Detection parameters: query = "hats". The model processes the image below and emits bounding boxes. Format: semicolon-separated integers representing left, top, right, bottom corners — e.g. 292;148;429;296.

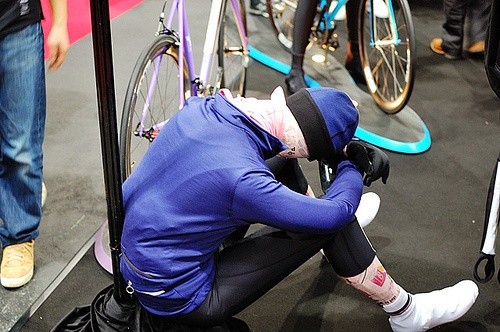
286;86;360;163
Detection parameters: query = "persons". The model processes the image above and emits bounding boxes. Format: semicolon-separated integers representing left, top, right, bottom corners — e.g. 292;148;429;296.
120;87;479;332
285;0;493;95
0;0;71;288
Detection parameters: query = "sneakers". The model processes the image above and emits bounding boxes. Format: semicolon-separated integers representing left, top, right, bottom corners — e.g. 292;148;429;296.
388;280;479;332
467;41;486;52
0;240;34;288
431;38;457;60
354;192;380;227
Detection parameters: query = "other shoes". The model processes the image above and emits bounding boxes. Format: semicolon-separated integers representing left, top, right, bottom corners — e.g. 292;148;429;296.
342;41;369;86
285;51;312;93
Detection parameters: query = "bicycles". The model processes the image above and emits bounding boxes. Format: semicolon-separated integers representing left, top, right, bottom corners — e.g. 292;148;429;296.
266;0;416;114
118;0;250;184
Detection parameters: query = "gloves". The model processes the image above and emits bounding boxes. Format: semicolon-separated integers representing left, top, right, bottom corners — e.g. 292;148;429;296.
347;139;390;186
